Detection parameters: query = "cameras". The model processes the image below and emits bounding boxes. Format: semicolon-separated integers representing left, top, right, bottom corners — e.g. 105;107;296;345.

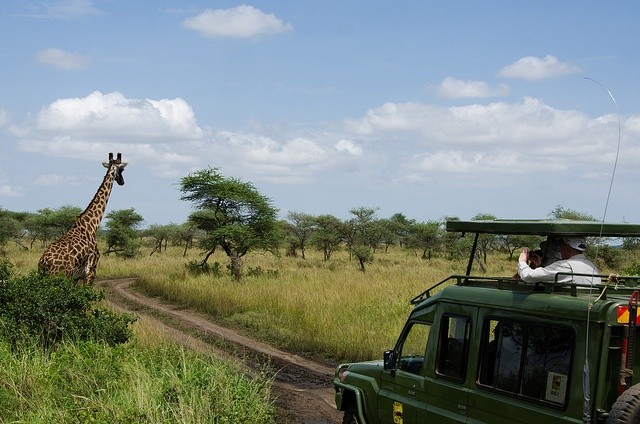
527;250;540;267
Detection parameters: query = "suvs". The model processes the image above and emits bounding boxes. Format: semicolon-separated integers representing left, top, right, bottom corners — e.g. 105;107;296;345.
332;76;640;424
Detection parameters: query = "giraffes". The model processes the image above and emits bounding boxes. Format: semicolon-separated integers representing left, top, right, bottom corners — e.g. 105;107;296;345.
38;153;129;285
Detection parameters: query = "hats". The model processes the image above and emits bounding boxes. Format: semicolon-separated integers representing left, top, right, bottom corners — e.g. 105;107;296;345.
552;235;587;252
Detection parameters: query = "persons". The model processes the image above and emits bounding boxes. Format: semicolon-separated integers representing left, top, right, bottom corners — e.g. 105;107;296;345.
516;235;601;290
